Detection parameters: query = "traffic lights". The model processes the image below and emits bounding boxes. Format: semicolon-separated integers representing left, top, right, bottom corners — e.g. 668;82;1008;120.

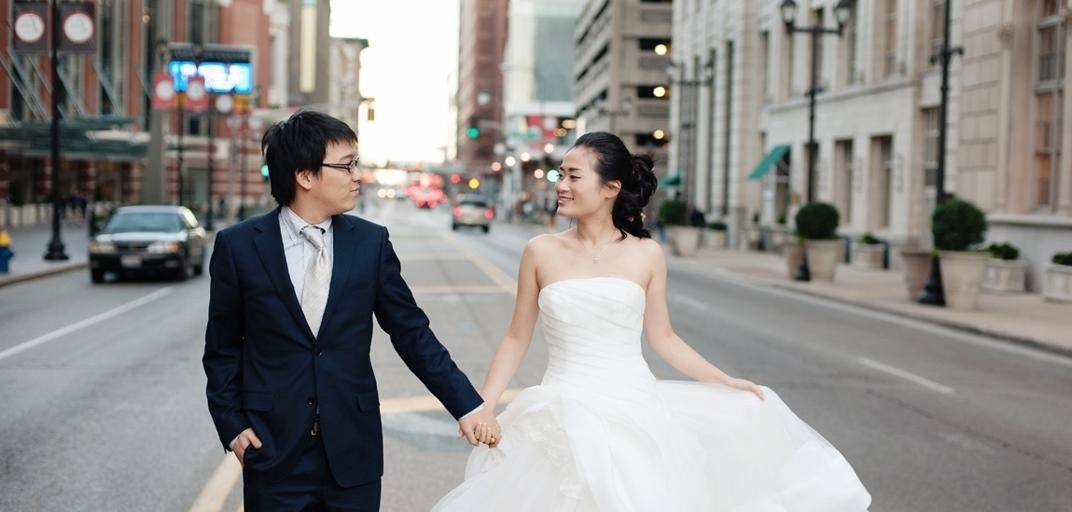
466;116;480;138
260;159;269;183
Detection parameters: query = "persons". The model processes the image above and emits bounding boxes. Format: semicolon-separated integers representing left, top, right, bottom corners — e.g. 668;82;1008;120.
203;111;495;512
431;131;870;512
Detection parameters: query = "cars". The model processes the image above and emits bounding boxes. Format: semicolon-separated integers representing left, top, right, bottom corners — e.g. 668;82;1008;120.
451;192;491;233
90;203;206;288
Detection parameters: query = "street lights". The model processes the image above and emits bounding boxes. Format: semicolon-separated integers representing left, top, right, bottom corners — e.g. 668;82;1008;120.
14;0;95;261
156;69;258;228
664;59;718;227
779;2;855;199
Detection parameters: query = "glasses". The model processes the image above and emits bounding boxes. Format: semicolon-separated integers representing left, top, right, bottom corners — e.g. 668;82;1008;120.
321;156;360;173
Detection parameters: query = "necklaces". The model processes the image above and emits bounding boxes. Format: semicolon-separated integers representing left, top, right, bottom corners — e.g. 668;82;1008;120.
573;230;623;267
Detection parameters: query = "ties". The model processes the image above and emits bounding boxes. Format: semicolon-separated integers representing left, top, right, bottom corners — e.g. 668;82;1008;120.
301;225;333;340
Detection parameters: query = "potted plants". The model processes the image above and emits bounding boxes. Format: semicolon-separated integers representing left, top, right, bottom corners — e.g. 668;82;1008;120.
972;238;1031;296
655;200;728;258
853;233;890;273
929;193;994;308
794;198;847;285
1039;251;1072;303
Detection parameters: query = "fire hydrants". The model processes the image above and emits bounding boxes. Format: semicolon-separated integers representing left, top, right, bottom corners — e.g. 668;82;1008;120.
0;230;15;276
